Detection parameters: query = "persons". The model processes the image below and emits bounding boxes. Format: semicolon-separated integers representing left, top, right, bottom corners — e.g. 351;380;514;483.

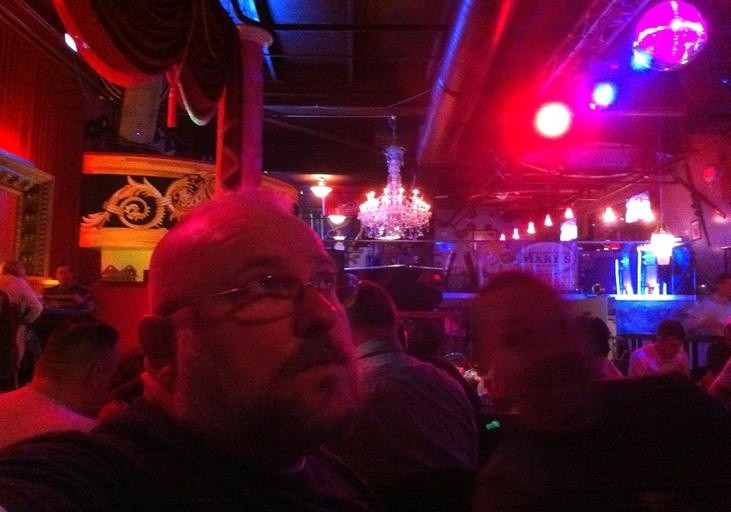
0;254;119;447
348;268;731;512
87;191;371;510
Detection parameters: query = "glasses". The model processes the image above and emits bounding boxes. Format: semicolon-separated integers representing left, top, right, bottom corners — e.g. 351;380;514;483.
152;269;363;326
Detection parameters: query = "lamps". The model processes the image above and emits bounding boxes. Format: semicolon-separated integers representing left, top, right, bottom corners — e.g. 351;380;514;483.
329;214;346;226
499;170;676;266
358;116;432;241
310;173;332;199
